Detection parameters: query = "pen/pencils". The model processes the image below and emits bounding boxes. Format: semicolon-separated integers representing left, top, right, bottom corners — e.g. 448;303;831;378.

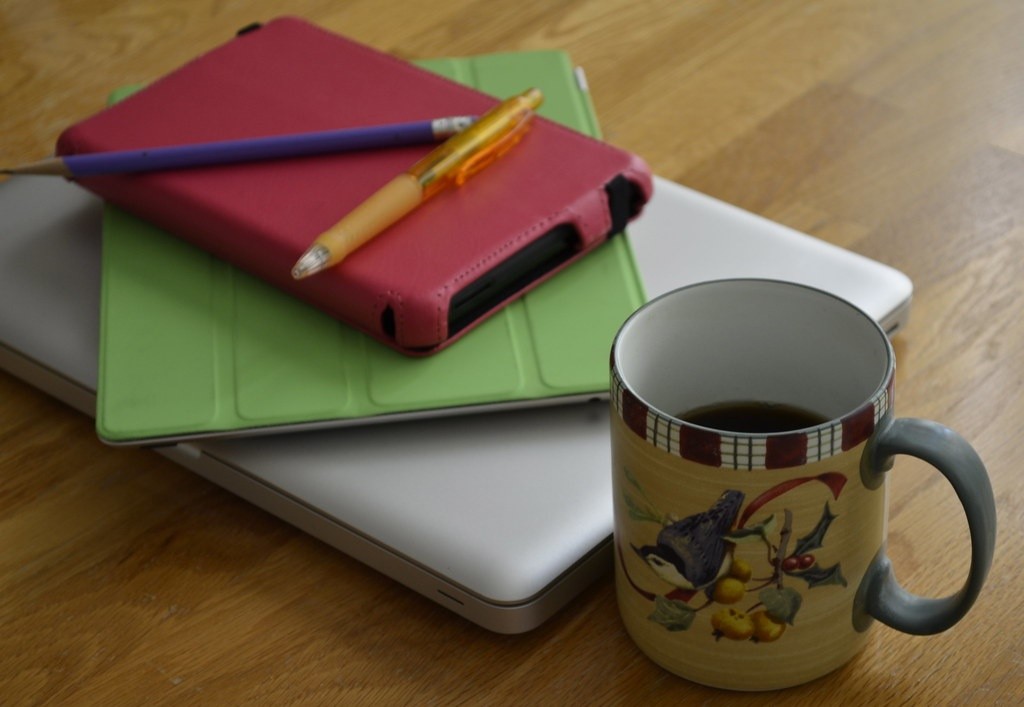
286;83;549;283
0;111;481;180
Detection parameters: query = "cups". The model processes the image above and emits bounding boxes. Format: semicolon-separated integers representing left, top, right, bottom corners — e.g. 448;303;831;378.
610;277;998;693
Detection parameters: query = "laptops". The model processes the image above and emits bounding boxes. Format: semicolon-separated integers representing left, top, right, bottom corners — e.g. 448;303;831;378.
1;172;913;634
